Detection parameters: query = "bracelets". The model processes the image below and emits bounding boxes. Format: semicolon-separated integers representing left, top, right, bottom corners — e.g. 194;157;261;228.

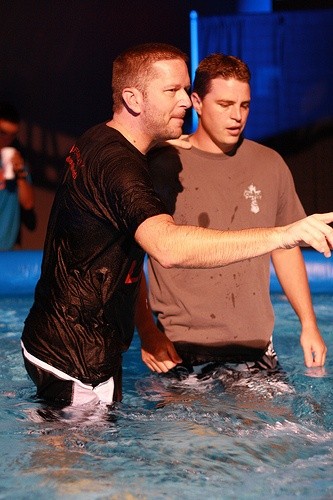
16;167;28;180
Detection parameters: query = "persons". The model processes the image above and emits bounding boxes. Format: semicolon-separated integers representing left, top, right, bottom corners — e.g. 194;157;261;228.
134;53;327;374
0;119;37;251
21;43;333;411
